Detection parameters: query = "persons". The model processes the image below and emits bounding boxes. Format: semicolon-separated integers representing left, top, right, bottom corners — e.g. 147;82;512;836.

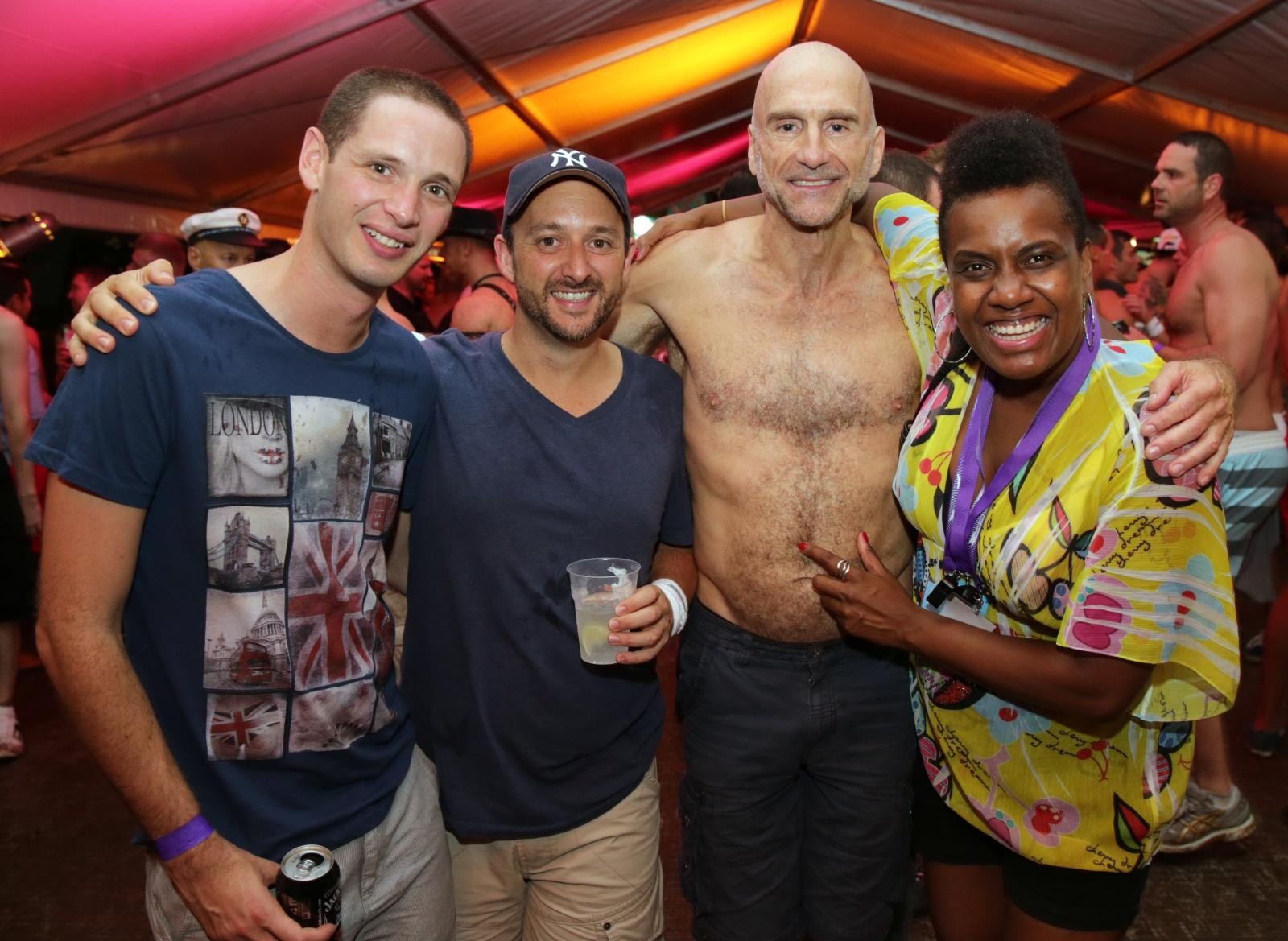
376;209;518;334
69;149;700;941
596;41;1238;941
23;67;474;941
1087;130;1288;854
634;106;1241;941
178;208;267;271
125;231;187;278
870;148;942;211
68;269;112;314
0;265;52;706
212;396;290;496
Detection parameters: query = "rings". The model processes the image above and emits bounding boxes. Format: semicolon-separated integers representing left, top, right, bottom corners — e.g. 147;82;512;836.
835;560;851;580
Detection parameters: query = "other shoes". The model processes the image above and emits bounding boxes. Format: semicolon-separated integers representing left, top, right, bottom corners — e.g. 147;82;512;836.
1241;634;1263;662
1249;725;1284;756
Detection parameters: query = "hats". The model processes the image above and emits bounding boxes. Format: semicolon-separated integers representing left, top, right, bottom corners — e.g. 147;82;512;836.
501;151;632;248
180;207;268;248
1151;228;1181;252
435;204;499;247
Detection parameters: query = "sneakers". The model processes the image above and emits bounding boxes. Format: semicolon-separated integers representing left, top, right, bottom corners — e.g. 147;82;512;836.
1157;782;1257;854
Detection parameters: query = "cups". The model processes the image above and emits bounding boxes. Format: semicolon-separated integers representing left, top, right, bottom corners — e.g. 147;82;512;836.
566;558;641;665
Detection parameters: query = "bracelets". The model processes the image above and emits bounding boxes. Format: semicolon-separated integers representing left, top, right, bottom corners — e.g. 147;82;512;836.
651;578;688;637
153;813;214;862
1154;343;1164;353
722;200;726;223
1146;317;1164;337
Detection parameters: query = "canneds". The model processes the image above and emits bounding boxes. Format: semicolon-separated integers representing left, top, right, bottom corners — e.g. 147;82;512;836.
275;843;341;941
